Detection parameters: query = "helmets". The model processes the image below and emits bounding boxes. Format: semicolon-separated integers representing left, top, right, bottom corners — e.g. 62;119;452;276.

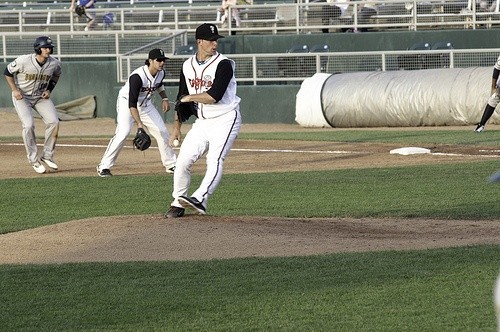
34;36;55;55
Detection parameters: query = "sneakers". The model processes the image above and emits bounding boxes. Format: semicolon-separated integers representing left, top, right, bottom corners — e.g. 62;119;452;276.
166;167;175;173
96;166;112;177
32;157;58;173
165;195;206;218
475;123;484;133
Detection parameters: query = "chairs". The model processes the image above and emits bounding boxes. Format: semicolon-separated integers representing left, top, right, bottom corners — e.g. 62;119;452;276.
169;0;457;78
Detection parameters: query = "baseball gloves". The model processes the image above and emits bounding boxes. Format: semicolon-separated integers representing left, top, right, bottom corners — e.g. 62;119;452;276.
175;94;194;123
132;128;151;151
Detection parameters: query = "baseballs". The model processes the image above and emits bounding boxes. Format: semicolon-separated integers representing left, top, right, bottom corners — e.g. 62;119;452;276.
173;140;179;147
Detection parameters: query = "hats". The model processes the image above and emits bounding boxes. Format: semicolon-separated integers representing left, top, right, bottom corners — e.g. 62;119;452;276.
195;23;225;41
149;48;169;61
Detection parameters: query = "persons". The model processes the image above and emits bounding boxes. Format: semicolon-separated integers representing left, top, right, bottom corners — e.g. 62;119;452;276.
70;0;99;38
95;49;178;179
220;0;242;30
474;54;500;132
361;0;378;25
164;23;242;218
4;36;64;175
321;0;351;33
